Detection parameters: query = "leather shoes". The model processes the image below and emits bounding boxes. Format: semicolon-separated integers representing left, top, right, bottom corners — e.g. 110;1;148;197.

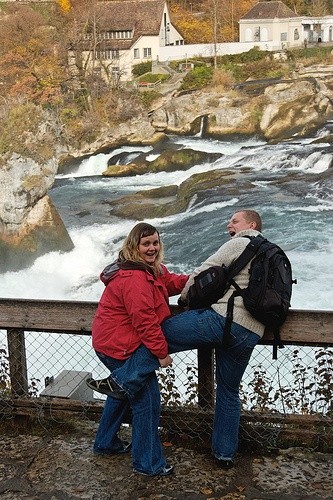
93;440;131;455
160;467;175;475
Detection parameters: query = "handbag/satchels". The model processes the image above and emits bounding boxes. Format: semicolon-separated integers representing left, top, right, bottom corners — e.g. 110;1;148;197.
185;265;233;310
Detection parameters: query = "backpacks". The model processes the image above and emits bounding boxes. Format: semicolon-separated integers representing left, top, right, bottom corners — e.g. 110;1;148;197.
241;234;291;327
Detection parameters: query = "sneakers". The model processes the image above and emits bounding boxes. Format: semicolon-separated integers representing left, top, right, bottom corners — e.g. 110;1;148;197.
213;456;233;469
86;376;126;400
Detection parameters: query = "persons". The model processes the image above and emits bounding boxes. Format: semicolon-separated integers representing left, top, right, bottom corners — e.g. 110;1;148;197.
90;222;192;476
85;209;295;470
